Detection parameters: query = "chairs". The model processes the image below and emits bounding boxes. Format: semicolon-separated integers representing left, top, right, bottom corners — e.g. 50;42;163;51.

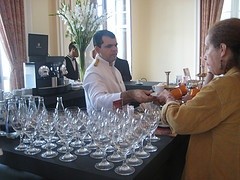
183;68;205;89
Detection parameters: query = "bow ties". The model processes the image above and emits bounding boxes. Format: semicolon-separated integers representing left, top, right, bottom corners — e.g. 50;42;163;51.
72;58;76;61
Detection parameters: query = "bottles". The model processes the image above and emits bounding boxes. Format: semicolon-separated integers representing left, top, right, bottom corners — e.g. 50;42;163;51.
0;89;64;139
49;66;59;87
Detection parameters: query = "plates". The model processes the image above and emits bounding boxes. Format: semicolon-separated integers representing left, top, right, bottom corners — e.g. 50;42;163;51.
150;92;163;97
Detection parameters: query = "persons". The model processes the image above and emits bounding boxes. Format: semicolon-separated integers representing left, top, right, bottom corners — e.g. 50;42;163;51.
62;41;133;82
83;30;157;113
153;18;240;180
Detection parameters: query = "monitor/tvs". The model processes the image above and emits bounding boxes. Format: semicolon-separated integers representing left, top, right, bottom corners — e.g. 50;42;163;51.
28;33;48;56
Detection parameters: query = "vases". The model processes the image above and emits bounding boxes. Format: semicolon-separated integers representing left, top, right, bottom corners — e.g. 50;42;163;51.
74;43;89;83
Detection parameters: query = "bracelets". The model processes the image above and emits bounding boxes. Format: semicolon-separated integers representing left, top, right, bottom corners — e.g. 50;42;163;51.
208;72;214;76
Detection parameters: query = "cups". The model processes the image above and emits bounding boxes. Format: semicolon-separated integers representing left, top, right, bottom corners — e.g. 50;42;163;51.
152;83;164;94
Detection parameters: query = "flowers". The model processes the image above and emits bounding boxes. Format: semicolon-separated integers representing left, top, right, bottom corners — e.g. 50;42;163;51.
48;0;110;82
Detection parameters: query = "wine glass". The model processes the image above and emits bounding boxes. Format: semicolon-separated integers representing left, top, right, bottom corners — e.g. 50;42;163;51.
11;107;162;175
